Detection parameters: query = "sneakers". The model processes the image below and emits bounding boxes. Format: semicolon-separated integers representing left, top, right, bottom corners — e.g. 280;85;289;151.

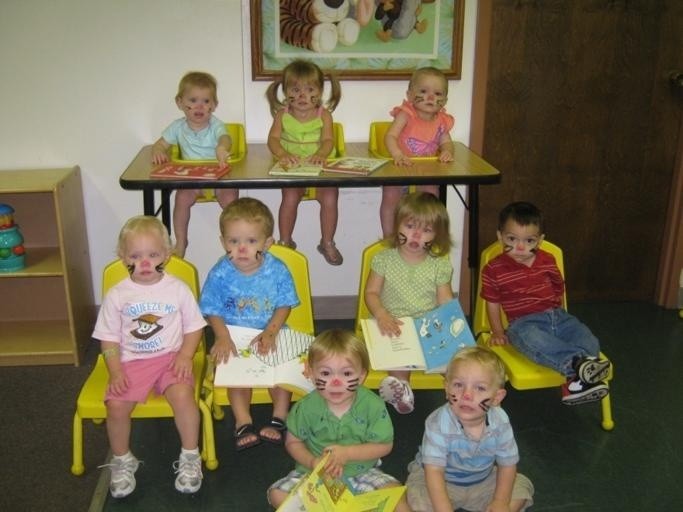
98;452;140;498
378;376;415;414
575;357;610;385
172;449;203;493
560;379;609;405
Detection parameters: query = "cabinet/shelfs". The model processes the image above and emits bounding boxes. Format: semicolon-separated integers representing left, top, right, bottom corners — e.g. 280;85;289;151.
0;167;95;371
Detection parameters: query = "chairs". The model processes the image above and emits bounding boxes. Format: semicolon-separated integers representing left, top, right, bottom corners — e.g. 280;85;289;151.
208;243;315;421
474;238;616;431
72;254;221;476
170;123;248;204
370;119;438;195
355;234;447;390
301;122;347;203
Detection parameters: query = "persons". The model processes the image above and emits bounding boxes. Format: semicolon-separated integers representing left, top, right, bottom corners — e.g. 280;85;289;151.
195;198;302;452
480;201;611;407
380;67;455;239
151;73;239;259
365;191;455;415
264;60;344;266
91;214;208;499
405;347;536;512
266;329;412;512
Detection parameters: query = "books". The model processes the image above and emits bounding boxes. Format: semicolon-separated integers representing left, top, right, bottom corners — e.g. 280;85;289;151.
359;297;479;374
274;452;408;512
150;163;232;180
269;154;389;176
214;323;316;397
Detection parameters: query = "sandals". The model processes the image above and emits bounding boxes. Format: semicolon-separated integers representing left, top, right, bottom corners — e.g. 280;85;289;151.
232;424;261;452
259;417;287;446
317;239;343;266
277;240;297;251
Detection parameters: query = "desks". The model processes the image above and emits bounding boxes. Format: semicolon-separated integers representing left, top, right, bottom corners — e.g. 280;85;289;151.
123;142;501;325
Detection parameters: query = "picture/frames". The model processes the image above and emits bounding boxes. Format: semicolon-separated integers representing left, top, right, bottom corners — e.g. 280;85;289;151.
250;0;467;81
